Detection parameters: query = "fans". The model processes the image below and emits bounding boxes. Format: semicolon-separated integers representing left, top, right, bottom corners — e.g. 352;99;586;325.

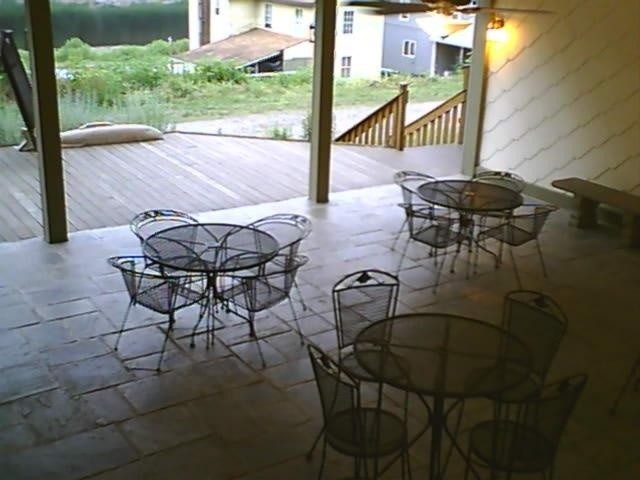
339;0;558;18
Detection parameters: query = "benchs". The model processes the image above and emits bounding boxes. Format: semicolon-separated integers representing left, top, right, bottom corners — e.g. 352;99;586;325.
551;177;640;251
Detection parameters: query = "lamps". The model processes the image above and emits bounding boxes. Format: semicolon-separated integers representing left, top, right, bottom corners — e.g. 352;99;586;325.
415;19;473;42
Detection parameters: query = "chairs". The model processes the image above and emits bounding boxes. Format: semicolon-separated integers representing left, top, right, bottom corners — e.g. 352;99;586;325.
304;268;412;463
305;342;412;480
465;371;588;479
444;286;567;479
107;209;313;374
389;170;559;294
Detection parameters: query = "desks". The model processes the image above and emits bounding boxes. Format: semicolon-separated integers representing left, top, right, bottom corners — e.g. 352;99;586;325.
353;313;534;480
